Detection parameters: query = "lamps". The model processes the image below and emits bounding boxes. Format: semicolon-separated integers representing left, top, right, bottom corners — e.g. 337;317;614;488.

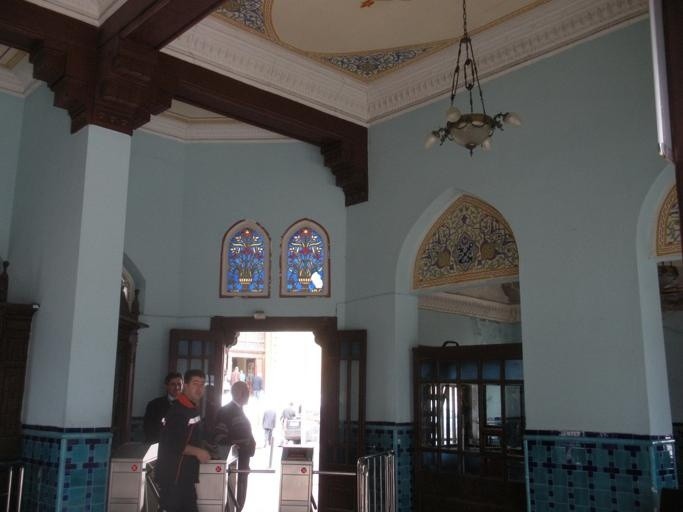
422;0;522;158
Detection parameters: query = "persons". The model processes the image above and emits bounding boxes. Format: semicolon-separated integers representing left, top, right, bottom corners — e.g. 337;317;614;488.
215;380;256;512
261;406;277;447
230;366;263;399
279;403;297;446
154;368;213;511
143;372;185;442
